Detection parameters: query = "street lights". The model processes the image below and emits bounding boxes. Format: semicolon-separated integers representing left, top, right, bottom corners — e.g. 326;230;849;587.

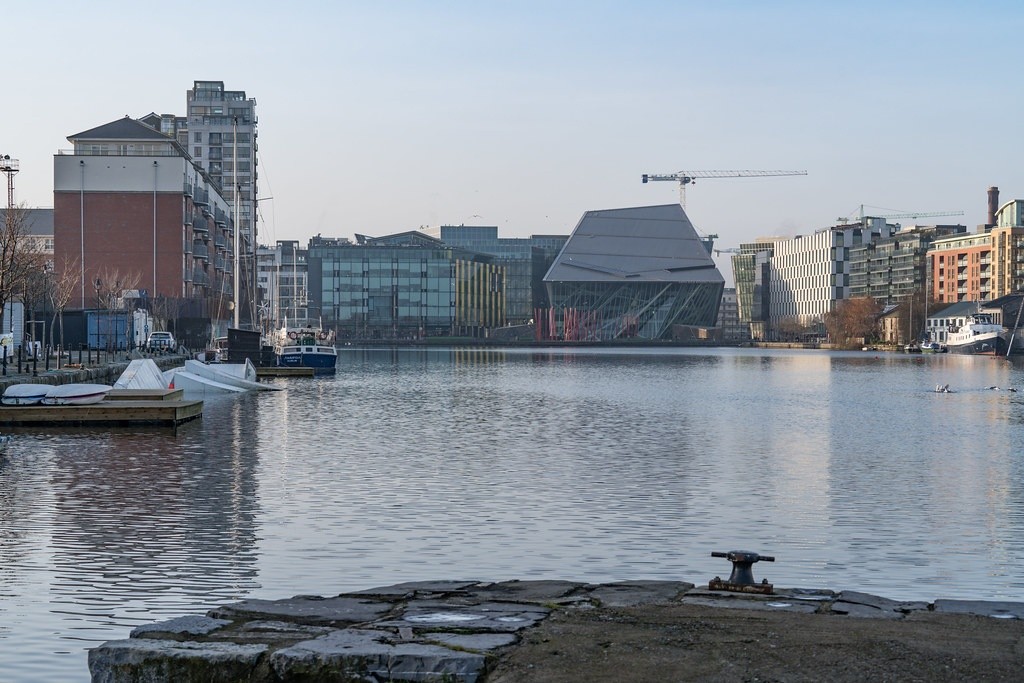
910;293;915;340
925;277;930;331
41;264;53;352
94;277;103;364
112;280;121;355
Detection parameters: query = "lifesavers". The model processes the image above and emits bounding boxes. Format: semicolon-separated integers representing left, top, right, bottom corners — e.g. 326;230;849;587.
289;331;298;340
319;332;327;340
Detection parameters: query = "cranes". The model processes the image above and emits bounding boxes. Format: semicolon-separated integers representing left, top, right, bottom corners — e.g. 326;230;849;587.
713;248;739;257
836;204;964;222
0;154;19;214
641;169;807;210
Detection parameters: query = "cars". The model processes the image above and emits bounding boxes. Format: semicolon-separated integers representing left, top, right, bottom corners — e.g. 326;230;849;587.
146;332;177;353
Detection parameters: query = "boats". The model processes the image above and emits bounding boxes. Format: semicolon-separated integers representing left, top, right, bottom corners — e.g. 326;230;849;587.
945;298;1007;355
920;341;945;352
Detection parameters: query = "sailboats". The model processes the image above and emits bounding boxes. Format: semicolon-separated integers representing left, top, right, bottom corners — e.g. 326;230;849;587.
198;185;337;374
